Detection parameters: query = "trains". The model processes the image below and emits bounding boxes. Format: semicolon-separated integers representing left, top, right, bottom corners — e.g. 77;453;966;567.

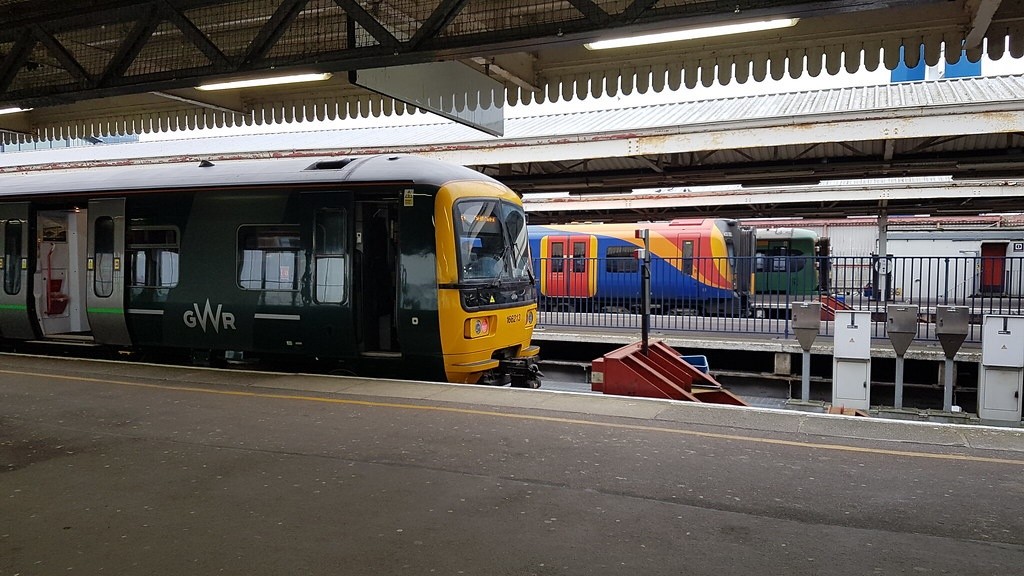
0;153;545;389
461;220;819;319
